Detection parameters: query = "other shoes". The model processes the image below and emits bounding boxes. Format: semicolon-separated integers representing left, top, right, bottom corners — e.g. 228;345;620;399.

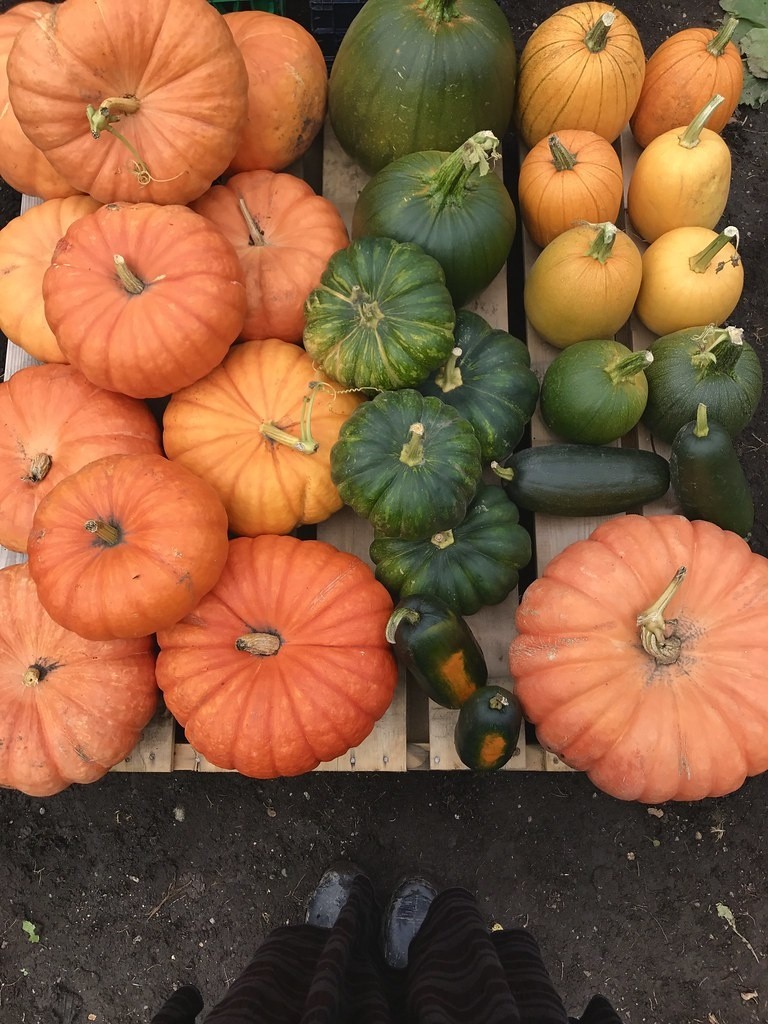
304;863;356;930
383;876;438;983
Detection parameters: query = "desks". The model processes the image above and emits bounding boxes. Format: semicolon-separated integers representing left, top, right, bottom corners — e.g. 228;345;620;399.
6;77;681;770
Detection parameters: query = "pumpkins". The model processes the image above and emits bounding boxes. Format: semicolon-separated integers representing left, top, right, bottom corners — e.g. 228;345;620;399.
0;0;768;804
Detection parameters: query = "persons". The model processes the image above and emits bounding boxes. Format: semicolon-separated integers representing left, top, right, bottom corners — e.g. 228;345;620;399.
146;862;625;1024
